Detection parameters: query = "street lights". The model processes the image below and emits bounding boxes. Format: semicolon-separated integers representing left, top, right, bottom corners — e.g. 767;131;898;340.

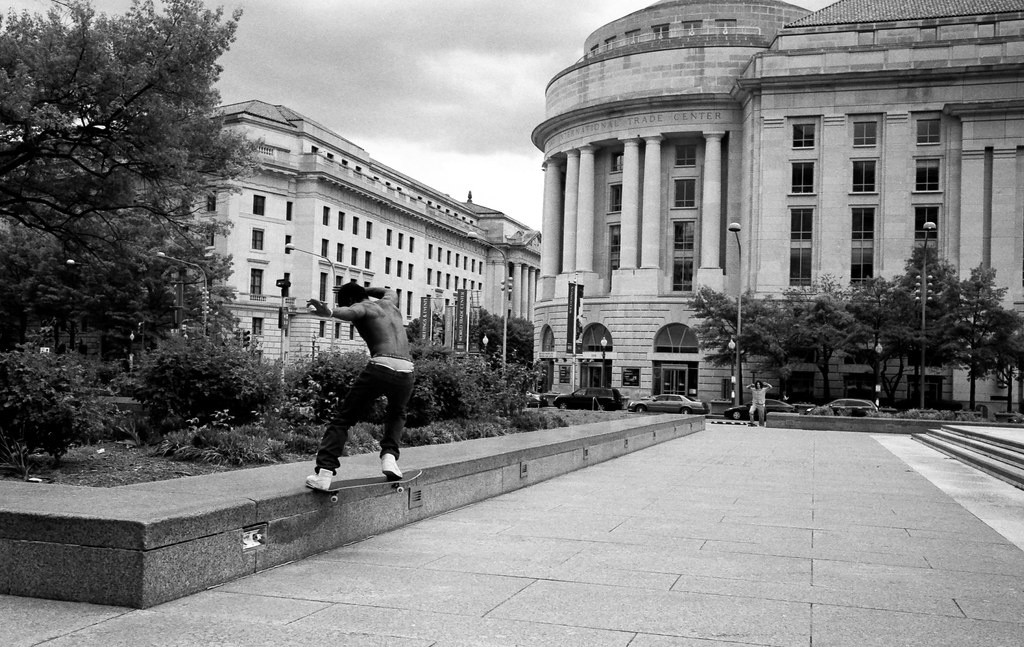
466;231;513;369
727;222;742;407
285;243;336;352
482;334;489;363
728;338;737;407
155;251;208;338
600;336;608;388
129;331;136;375
875;342;884;408
920;221;936;409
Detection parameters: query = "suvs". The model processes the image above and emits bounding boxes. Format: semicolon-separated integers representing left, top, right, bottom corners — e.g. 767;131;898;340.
553;386;624;412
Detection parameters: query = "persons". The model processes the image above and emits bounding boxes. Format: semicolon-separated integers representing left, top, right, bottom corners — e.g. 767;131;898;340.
778;390;790;404
746;381;772;426
306;282;415;490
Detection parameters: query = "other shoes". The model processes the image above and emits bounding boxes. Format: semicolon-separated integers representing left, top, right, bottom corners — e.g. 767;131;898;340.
381;453;403;480
305;467;333;492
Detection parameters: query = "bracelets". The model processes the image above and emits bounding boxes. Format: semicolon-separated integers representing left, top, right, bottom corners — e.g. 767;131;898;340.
326;308;333;318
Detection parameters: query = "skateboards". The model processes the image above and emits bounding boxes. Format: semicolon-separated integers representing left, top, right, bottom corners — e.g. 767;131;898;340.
305;468;423;504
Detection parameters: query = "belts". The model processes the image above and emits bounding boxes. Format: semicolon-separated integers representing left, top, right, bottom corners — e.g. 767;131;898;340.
368;362;414;379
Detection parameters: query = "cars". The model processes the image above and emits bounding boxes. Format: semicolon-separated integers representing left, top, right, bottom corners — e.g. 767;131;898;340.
805;398;878;417
627;394;711;415
724;398;800;420
